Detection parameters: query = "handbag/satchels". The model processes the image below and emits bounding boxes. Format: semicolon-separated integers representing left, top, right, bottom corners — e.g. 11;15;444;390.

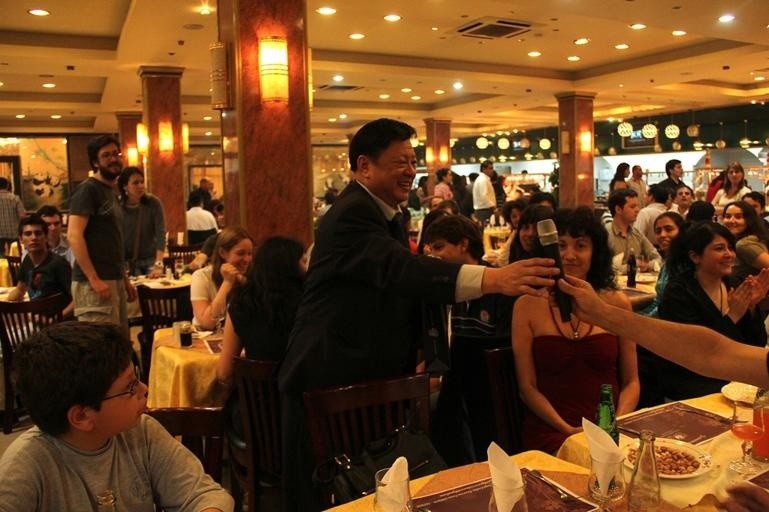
312;424;448;505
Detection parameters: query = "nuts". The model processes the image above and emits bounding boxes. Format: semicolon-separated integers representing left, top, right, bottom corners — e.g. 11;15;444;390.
627;445;699;475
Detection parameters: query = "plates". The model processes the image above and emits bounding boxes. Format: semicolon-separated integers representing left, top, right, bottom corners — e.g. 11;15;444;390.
619;437;715;480
626;275;658;283
720;380;759;405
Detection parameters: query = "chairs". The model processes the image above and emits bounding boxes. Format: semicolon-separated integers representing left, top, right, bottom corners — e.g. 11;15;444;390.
0;214;769;511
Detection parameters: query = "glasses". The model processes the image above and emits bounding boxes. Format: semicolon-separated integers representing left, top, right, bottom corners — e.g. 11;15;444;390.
101;366;141;402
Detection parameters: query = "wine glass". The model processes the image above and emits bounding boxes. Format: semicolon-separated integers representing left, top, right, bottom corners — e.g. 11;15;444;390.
732;399;763;474
588;453;626;512
174;259;184;281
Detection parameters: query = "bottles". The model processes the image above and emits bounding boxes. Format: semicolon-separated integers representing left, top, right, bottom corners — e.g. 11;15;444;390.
626;247;637;288
167;268;172;280
627;430;660;510
596;383;620;450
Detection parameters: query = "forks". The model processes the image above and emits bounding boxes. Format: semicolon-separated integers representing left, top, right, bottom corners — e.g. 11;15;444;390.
672;406;729;422
520;467;582;503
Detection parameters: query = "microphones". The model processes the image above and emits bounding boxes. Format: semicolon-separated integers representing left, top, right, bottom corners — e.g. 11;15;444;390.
536;216;578;325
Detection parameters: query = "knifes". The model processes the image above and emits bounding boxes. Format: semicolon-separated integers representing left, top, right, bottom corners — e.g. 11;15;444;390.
533;469;598;507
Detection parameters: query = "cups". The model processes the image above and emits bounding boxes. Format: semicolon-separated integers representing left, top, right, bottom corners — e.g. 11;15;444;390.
173;321;193;348
148;267;160;280
751;386;769;463
637;255;649;272
373;468;413;512
485;478;530;511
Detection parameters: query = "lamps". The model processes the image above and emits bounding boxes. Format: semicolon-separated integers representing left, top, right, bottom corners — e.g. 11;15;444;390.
127;121;190;167
207;40;233;110
305;45;316;112
475;116;754;159
258;30;290;106
